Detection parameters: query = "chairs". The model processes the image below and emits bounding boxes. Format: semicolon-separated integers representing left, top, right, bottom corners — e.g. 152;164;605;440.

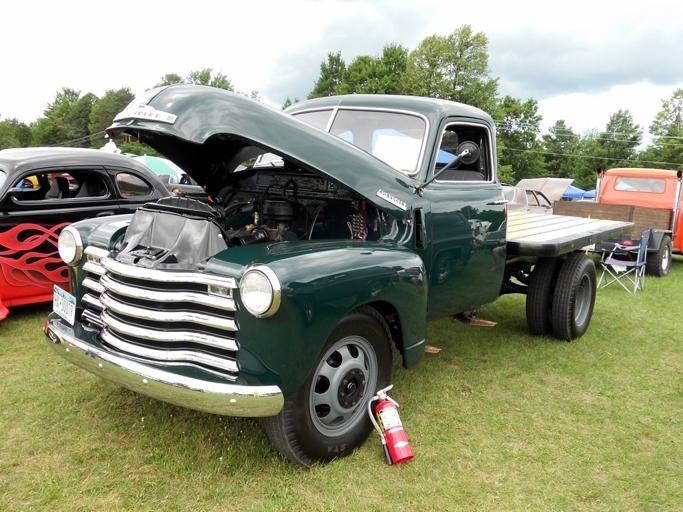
44;176;68;199
595;228;652;295
76;180;98;195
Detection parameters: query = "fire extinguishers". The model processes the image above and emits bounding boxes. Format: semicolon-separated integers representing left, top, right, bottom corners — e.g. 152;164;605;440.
368;384;415;464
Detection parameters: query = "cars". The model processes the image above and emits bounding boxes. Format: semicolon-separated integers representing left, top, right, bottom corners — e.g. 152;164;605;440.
0;141;182;320
